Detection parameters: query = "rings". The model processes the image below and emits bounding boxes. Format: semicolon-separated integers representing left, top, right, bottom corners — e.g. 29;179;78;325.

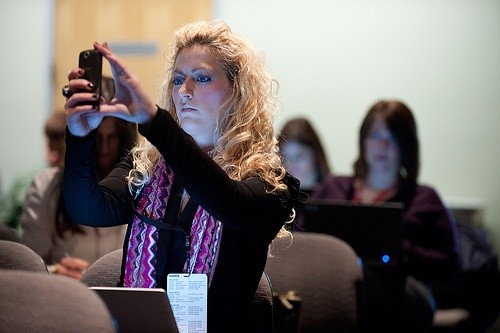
62;85;72;97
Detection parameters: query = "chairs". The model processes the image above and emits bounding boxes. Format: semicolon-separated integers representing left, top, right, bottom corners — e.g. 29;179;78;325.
0;230;369;333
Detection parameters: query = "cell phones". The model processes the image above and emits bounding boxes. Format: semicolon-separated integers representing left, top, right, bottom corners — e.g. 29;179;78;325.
79;50;103;108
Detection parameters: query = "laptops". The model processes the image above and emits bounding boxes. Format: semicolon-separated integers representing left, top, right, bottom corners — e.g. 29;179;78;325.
303;201;409;297
88;286;182;333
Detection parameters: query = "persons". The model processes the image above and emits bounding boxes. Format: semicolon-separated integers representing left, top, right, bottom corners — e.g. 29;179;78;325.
293;101;463;280
20;110;68;231
278;118;332;190
21;77;139;280
60;20;311;333
